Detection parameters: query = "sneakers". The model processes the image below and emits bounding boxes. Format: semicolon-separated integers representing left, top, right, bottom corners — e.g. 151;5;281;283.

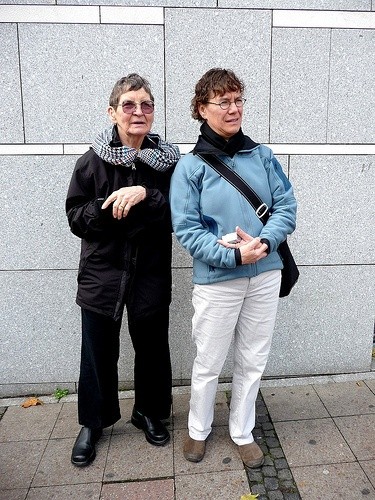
182;436;205;462
237;442;265;470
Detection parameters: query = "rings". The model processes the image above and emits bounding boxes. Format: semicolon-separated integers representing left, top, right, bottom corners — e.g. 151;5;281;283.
120;204;124;208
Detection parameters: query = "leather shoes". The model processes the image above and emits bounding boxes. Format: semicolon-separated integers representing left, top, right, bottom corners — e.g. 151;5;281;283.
130;408;170;447
71;427;103;467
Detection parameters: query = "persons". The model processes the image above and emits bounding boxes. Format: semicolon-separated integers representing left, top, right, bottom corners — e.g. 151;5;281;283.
65;73;179;466
169;67;297;468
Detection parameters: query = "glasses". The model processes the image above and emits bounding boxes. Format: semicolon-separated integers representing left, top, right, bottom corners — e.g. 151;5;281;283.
206;98;246;109
112;100;154;115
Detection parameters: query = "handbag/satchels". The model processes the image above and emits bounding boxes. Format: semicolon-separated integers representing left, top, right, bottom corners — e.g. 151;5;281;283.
276;239;300;298
